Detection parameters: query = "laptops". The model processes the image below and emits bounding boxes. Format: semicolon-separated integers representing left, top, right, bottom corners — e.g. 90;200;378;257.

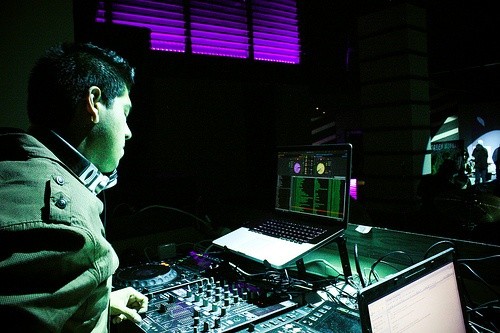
211;143;352;269
360;247;472;333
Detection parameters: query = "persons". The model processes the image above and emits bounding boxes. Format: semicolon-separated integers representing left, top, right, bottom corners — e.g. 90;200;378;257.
0;42;148;333
492;147;500;180
437;153;460;173
473;144;488;184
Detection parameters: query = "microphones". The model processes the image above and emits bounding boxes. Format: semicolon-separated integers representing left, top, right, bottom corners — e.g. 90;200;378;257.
136;205;231;238
